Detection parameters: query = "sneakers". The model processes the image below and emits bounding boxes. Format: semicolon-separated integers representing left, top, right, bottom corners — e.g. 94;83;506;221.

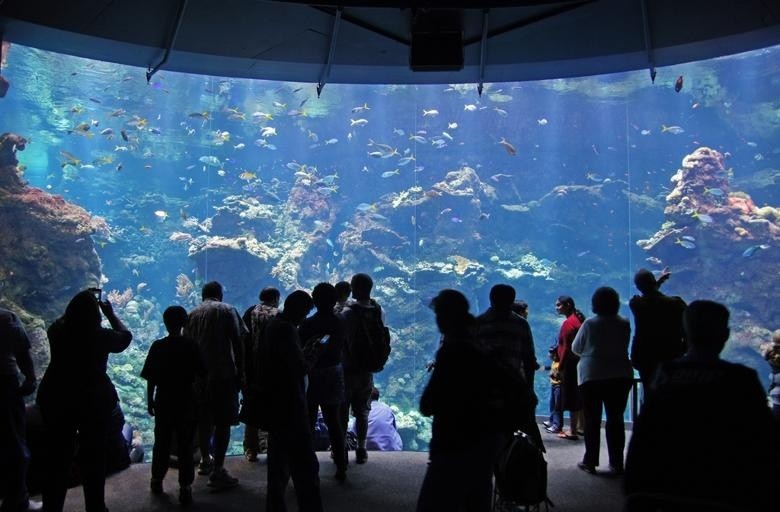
355;447;369;464
150;478;164;493
245;448;257;461
577;461;596;473
576;428;585;436
207;470;239;489
178;487;193;504
543;420;562;433
333;464;348;481
608;462;624;473
198;459;215;475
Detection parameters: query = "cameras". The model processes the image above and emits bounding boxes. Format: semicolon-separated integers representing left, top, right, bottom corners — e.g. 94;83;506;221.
89;289;101;301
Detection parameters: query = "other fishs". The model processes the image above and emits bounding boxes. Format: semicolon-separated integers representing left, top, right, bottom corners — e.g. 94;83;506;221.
541;257;558;268
41;60;548;251
136;282;148;294
583;67;773;282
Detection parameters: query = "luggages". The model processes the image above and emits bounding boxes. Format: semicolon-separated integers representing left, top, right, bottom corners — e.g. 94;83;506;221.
492;425;549;511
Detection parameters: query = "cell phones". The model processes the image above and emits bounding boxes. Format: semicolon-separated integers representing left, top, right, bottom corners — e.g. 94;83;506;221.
320;334;331;344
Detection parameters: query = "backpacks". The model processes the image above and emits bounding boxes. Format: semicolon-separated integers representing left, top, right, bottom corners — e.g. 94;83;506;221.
343;301;390;375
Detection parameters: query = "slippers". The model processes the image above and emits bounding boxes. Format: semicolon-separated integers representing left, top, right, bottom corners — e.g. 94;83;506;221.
555;431;580;440
